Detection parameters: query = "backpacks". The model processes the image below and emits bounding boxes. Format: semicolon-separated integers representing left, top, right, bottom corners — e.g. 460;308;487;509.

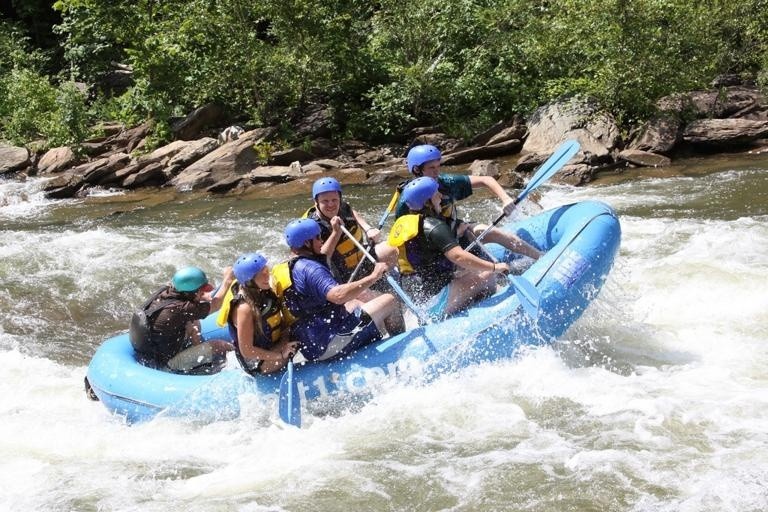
128;311;149;350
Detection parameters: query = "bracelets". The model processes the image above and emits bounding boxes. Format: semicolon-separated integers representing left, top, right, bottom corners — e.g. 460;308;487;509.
281;351;285;360
366;227;375;234
494;262;495;273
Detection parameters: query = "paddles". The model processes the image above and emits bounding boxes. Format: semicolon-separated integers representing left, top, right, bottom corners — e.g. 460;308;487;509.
463;140;580;254
279;336;301;428
466;228;540;320
341;225;463;362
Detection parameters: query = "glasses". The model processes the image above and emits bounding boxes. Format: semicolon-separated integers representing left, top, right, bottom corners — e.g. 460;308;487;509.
317;234;321;240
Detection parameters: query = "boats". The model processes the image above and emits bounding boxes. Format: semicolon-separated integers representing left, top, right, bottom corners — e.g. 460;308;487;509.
85;200;621;431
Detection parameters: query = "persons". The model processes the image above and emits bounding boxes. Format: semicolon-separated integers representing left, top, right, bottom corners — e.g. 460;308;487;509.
225;253;304;378
387;176;510;323
129;266;236;372
395;144;545;260
302;177;399;302
275;215;405;362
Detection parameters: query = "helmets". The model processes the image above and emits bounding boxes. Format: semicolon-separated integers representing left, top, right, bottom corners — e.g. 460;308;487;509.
400;176;439;211
284;217;322;249
232;253;268;285
171;266;208;293
406;144;442;173
312;176;343;199
